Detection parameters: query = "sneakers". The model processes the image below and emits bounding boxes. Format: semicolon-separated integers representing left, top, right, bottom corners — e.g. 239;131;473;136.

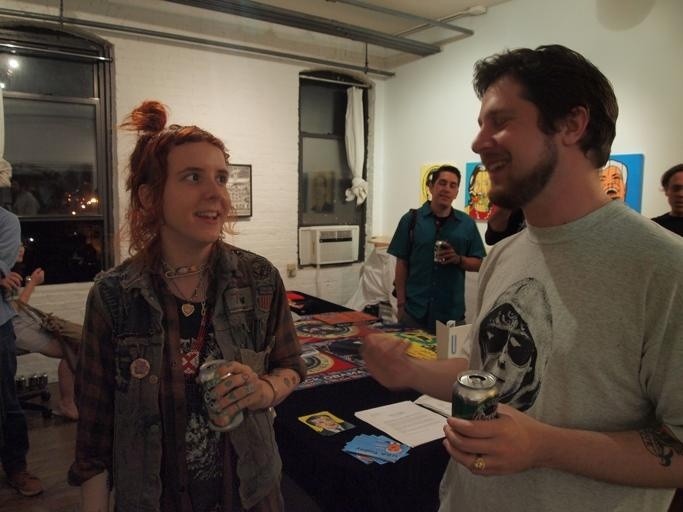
1;470;45;496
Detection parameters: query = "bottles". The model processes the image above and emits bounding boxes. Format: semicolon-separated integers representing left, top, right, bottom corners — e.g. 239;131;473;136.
14;371;48;391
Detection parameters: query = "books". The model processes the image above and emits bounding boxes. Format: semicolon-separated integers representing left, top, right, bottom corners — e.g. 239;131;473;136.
434;318;472;360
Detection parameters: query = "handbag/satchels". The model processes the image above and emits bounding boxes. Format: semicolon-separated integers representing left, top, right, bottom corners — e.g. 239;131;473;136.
42;311;82;342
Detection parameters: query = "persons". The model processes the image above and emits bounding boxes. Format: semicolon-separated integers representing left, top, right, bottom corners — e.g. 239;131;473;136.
648;164;682;239
481;198;528;247
64;98;307;511
0;202;47;502
351;42;682;512
597;159;631;204
0;174;41;217
475;275;553;412
5;240;84;421
464;164;498;221
306;414;356;437
385;164;489;336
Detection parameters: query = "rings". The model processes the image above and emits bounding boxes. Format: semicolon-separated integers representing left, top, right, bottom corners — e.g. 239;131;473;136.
473;455;486;472
240;371;250;384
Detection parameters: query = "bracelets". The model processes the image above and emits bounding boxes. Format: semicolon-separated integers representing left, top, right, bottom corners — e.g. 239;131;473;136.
259;376;277;410
396;302;406;308
456;255;463;267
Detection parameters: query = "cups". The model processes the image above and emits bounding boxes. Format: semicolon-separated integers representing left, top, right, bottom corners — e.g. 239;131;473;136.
25;275;32;285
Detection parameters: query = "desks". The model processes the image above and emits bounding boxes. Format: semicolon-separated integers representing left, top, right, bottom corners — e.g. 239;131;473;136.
272;291;450;512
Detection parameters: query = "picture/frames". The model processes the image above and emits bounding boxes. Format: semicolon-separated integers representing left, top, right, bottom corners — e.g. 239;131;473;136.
226;164;252;218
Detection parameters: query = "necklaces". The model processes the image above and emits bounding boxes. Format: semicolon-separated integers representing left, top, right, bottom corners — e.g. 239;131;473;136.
154;249;212;319
